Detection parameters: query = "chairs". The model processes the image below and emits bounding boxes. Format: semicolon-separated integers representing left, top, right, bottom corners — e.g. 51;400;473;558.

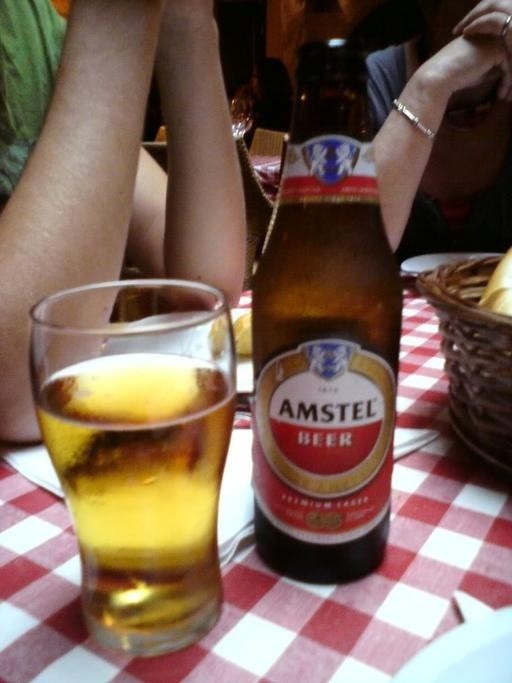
143;140;274;297
248;126;289;156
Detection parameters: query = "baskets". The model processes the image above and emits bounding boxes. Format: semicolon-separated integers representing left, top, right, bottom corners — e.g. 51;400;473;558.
415;253;511;480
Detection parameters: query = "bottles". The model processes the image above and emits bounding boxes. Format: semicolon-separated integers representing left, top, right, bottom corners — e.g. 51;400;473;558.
251;38;404;589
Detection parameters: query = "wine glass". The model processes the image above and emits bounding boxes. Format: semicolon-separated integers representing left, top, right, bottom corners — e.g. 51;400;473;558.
229;96;254;141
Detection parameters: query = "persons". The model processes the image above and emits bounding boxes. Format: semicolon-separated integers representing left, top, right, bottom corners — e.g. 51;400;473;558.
2;2;248;443
368;0;510;254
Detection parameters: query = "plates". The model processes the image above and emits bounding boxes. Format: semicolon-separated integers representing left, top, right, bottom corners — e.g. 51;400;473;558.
400;252;504;274
100;309;257;394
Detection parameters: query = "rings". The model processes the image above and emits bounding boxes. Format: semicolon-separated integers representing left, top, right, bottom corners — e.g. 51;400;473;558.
500;11;511;40
391;98;436;143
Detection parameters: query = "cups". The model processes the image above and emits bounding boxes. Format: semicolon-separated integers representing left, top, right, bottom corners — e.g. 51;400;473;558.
30;276;237;660
